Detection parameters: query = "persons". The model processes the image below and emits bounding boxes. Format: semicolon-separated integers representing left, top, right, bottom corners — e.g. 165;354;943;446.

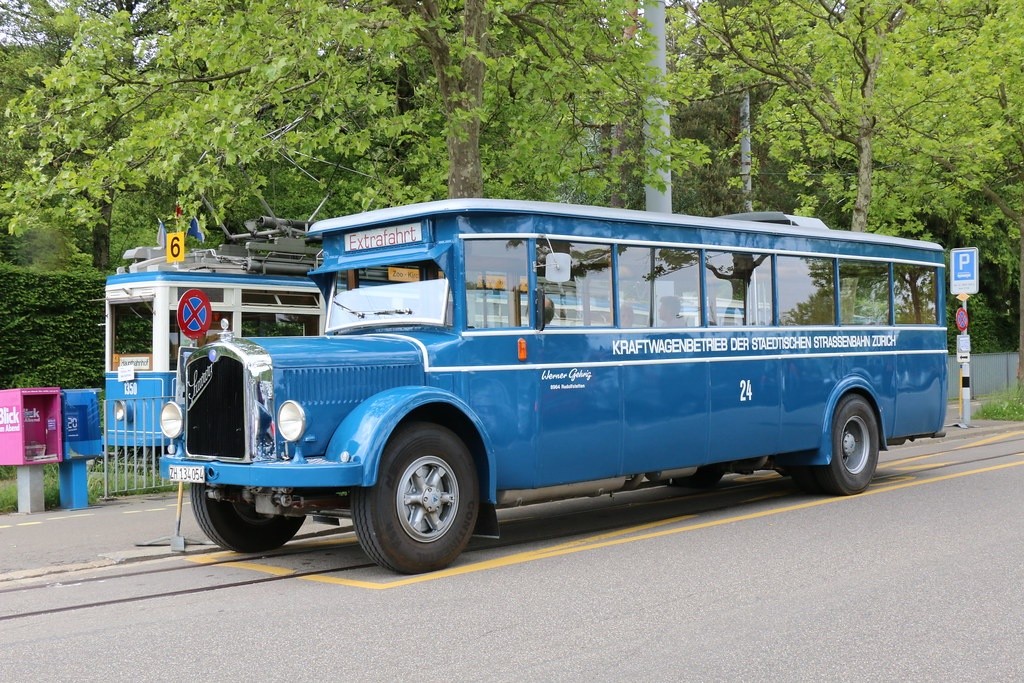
658;297;685;327
620;301;637;328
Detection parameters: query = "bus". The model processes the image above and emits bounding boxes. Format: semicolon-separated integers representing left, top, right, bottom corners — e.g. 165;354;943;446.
159;195;951;576
